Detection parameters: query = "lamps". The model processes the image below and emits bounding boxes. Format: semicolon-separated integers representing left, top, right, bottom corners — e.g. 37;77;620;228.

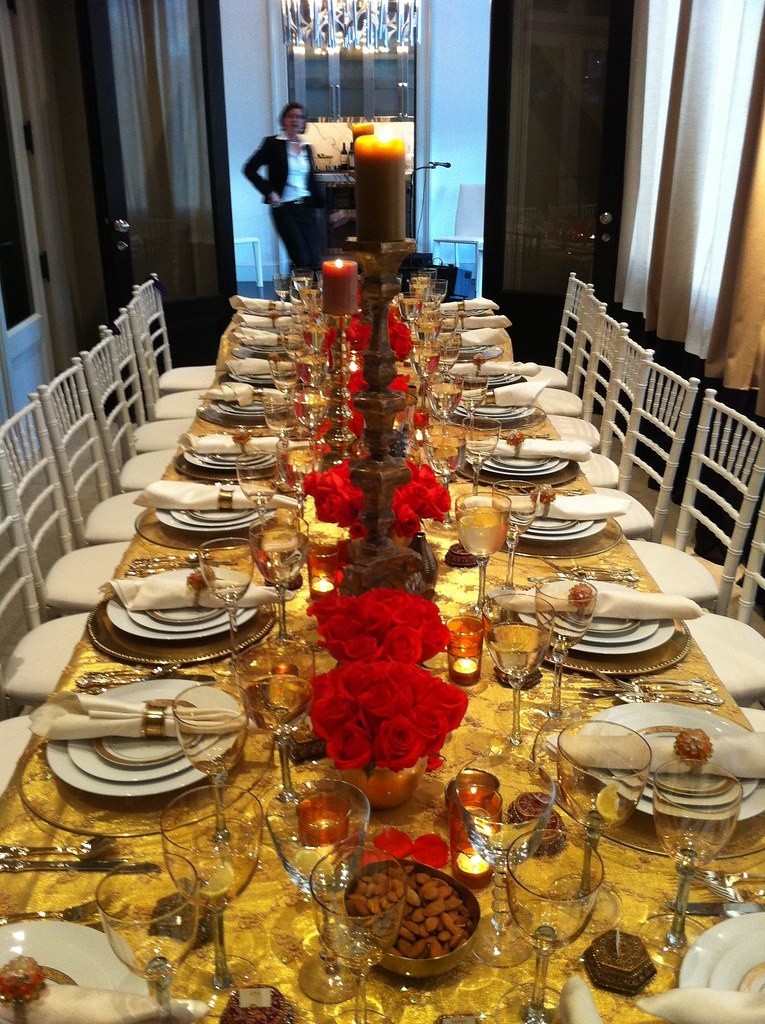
281;0;422;49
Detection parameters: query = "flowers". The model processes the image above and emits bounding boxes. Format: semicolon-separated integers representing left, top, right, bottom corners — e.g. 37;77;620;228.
303;301;468;769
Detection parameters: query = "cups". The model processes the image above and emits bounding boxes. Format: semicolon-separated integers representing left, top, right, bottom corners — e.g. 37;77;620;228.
306;544;339;601
444;616;485;684
450;786;503;888
297;794;352;848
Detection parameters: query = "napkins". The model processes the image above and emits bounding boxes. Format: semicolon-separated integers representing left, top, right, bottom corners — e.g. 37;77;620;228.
0;979;211;1024
439;296;702;621
560;972;764;1024
28;690;244;740
98;295;292;615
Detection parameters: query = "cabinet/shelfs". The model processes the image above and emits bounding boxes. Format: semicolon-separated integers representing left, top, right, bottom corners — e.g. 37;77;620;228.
286;36;416;123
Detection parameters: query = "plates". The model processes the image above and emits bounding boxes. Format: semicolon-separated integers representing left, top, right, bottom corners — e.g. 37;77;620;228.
432;292;765;1024
1;292;300;1024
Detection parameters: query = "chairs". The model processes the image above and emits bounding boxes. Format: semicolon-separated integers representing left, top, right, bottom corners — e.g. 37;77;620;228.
433;185;485;298
0;450;93;720
522;272;765;618
0;272;216;614
685;497;765;706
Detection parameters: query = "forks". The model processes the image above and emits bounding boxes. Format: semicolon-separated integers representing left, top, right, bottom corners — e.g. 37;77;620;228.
0;898;108;922
704;882;764;902
676;860;765;885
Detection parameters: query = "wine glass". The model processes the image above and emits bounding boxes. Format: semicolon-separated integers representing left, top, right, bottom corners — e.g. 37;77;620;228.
91;252;743;1015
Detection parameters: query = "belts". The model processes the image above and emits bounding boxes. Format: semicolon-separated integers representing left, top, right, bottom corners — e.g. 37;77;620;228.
282;199;310;204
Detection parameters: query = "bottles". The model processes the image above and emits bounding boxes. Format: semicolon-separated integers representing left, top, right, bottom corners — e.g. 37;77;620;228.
407;532;438;589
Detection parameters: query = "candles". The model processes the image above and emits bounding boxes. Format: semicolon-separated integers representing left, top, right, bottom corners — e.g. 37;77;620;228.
352;123;374;143
354;136;406;240
322;260;358;315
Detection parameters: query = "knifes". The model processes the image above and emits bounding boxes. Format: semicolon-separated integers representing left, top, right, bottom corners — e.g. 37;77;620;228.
668;901;764;914
75;674;217;687
1;860;162;873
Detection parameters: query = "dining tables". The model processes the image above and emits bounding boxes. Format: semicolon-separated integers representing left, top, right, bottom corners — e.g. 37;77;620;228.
543;732;765;780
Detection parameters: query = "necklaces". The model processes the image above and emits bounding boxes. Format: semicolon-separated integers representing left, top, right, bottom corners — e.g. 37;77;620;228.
292;141;299;144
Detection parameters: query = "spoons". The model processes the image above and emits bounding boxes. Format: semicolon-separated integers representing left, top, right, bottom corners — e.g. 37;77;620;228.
0;836;117;859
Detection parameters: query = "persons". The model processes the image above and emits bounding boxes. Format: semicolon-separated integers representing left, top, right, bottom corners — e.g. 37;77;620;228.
241;102;326;277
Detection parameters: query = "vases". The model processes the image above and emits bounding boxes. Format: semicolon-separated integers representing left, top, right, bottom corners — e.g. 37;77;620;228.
342;757;428;810
354;534;413;549
